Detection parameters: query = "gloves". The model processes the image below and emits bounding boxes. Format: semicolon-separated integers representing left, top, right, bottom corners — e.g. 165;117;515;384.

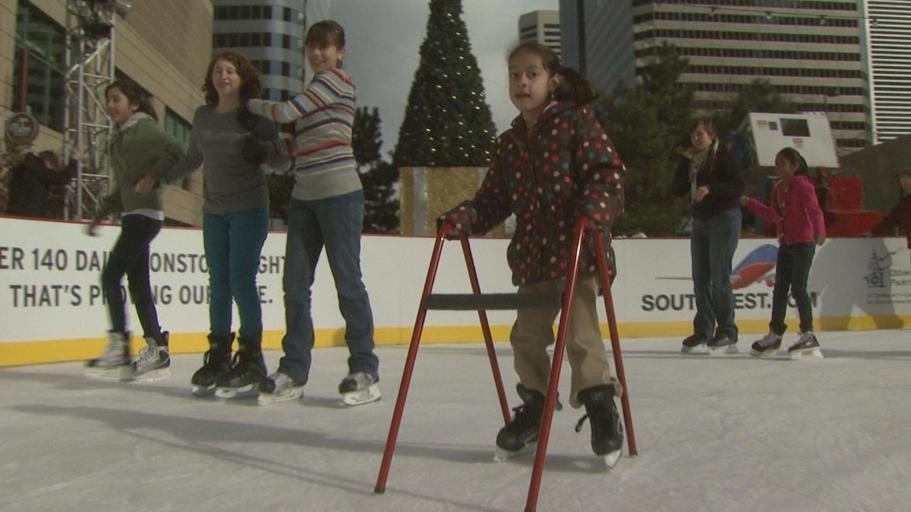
242;143;266;164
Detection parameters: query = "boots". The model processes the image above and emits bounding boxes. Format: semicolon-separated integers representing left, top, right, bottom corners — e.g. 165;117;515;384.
192;332;234;387
575;385;623;456
87;329;130;368
751;323;787;352
495;383;545;452
215;338;265;389
788;326;819;352
129;331;169;376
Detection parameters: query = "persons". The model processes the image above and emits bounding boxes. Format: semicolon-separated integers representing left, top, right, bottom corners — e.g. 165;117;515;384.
151;53;294;400
239;18;387;407
863;169;911;250
670;116;743;355
5;149;80;222
83;80;186;386
739;146;827;362
437;41;630;465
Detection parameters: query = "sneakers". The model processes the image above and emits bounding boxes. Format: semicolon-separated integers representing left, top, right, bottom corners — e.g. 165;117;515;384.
338;372;377;394
259;371;292;395
682;333;706;347
707;336;736;347
